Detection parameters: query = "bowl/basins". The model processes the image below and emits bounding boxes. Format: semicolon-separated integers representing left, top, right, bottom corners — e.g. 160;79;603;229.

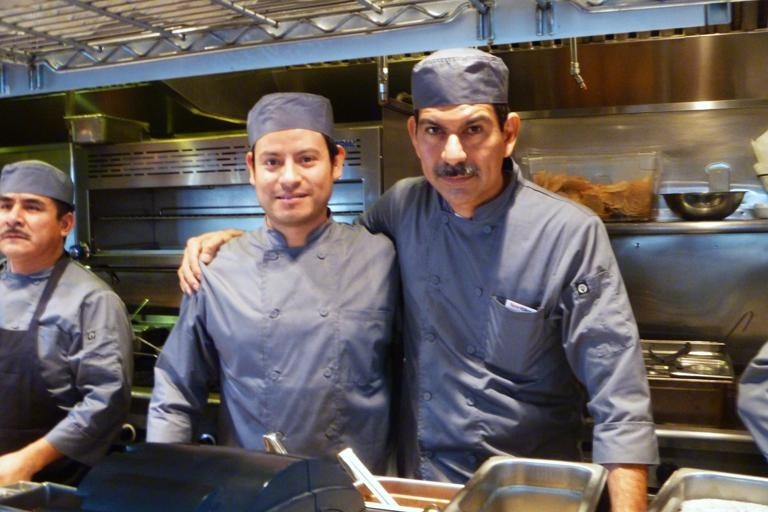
658;191;746;220
745;208;767;219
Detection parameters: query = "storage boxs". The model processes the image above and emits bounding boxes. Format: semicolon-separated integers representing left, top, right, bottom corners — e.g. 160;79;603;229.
519;149;663;222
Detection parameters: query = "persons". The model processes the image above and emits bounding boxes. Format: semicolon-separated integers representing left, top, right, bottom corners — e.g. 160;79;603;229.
175;46;662;509
736;342;767;462
148;91;403;476
1;155;130;491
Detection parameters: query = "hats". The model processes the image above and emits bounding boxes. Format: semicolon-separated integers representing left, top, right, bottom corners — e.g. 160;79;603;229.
247;92;333;150
0;160;73;204
410;48;510;111
